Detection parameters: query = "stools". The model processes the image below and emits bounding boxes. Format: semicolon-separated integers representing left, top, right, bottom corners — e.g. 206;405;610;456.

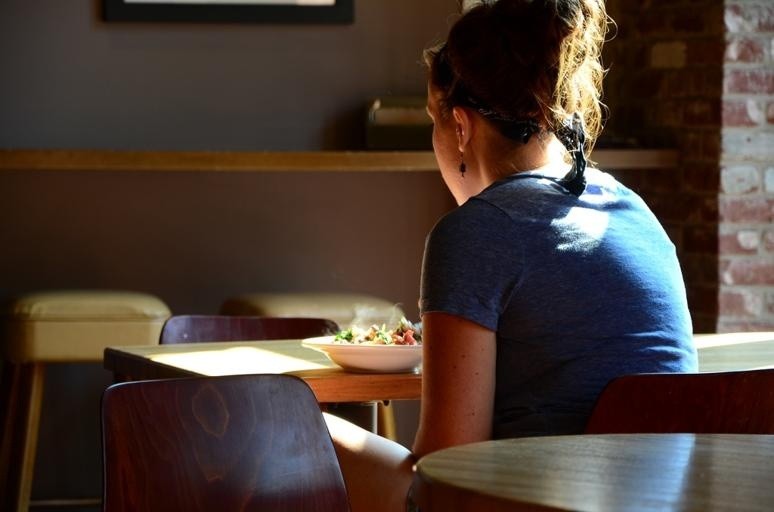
222;291;408;445
10;289;176;510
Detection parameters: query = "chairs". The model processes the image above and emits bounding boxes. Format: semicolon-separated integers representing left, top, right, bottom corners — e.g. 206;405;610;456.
581;370;772;435
102;376;352;511
162;315;339;342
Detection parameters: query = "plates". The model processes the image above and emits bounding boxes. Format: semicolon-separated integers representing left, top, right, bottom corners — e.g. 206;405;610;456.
303;335;424;371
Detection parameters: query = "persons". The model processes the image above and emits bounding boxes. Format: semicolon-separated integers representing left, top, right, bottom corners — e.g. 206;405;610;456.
320;1;696;512
